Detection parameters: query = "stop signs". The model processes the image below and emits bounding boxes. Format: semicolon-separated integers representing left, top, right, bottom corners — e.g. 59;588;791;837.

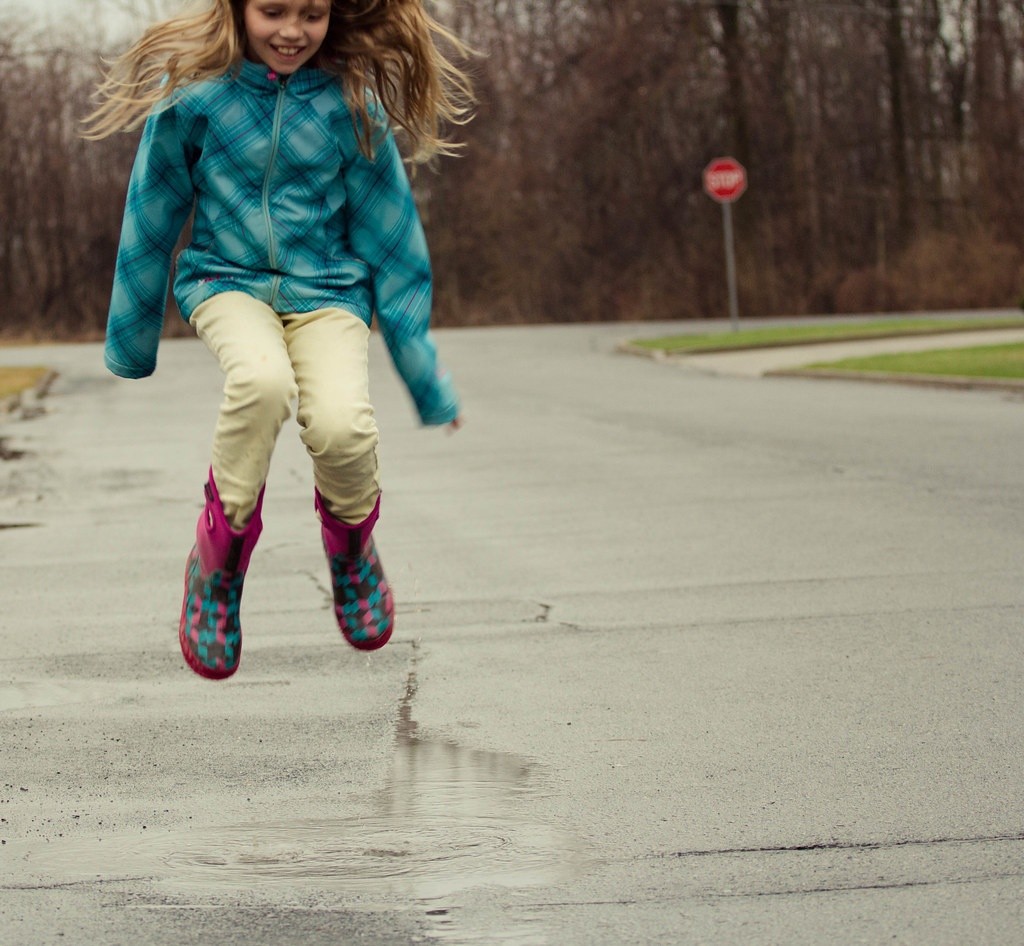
702;156;747;205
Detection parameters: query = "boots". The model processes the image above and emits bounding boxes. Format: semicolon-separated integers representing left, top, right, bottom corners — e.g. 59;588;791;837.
314;484;394;651
179;464;267;680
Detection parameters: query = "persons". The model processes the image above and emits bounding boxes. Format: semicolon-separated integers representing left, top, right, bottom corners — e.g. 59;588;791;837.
76;0;490;683
